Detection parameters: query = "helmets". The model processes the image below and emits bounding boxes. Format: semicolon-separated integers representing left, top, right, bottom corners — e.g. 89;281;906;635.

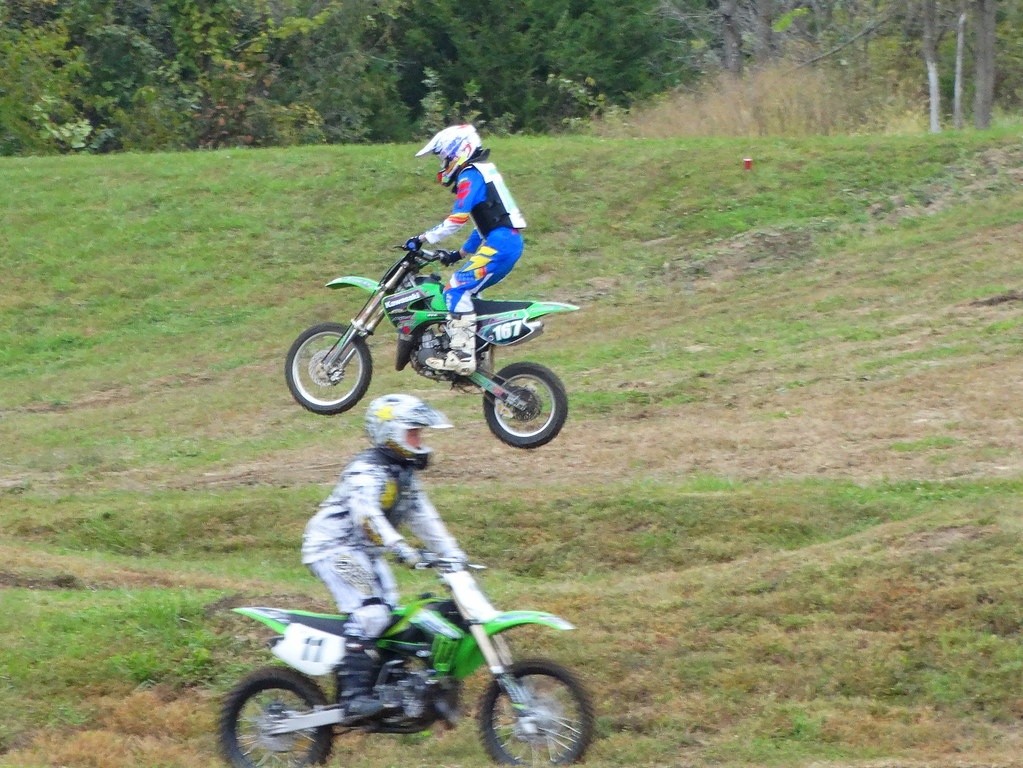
361;392;455;471
414;124;484;188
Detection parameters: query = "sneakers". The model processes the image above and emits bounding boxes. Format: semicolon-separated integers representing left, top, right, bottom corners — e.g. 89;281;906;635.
340;692;402;728
424;349;477;377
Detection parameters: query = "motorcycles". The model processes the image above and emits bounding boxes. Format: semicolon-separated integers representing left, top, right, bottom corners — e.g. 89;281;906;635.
214;551;594;768
285;241;580;451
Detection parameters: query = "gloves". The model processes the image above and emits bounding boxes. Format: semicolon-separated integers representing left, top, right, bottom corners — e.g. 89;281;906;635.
435;249;462;267
402;235;424;251
399;547;441;571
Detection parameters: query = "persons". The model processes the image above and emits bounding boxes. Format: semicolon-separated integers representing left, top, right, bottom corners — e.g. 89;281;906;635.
405;124;526;376
301;394;467;717
